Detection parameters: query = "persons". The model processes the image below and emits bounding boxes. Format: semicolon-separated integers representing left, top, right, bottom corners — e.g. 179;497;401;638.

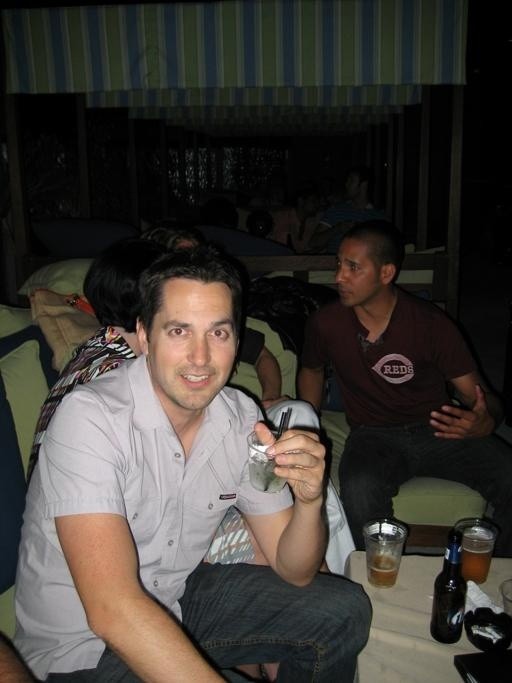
166;231;293;410
305;161;391;255
295;225;511;558
264;171;290;210
254;178;326;256
25;247;160;491
10;249;373;683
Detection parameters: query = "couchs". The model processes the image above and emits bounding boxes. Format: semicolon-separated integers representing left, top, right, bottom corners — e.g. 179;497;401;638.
0;304;487;682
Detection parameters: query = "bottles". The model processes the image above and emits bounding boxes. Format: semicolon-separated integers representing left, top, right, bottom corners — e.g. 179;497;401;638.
429;530;466;645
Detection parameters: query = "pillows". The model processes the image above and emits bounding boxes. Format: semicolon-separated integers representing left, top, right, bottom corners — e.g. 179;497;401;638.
1;323;61;639
0;305;33;337
18;257;96;297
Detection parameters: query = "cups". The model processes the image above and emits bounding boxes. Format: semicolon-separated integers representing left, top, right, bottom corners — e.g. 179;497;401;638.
499;579;512;618
247;430;287;494
455;517;498;585
362;519;407;589
